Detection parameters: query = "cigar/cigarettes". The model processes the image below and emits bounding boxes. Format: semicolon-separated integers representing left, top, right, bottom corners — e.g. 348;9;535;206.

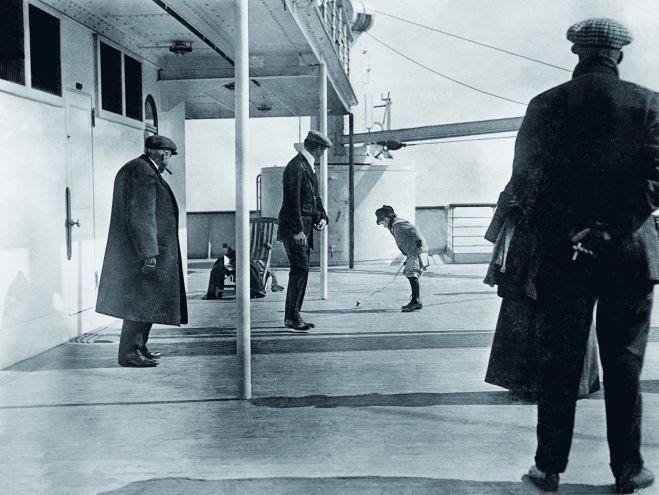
164;167;173;175
572;239;582;262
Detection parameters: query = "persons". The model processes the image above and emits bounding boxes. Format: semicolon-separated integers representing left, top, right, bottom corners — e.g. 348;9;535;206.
513;18;659;492
484;179;602;403
376;205;430;314
95;134;188;368
277;130;334;331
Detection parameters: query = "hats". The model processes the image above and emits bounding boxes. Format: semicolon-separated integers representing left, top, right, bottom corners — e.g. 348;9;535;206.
145;135;178;152
307;130;333;148
375;204;394;224
565;18;633;50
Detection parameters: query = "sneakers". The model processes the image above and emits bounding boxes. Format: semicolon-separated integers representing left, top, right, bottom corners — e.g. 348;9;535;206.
401;299;421;312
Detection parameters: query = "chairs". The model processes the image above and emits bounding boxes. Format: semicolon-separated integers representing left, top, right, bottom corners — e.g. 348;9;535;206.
225;213;285;296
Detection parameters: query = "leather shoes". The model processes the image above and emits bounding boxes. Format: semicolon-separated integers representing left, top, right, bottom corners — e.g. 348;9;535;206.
529;464;559;492
284;317;315;331
616;466;655;494
120;351;158;369
139;348;163;359
